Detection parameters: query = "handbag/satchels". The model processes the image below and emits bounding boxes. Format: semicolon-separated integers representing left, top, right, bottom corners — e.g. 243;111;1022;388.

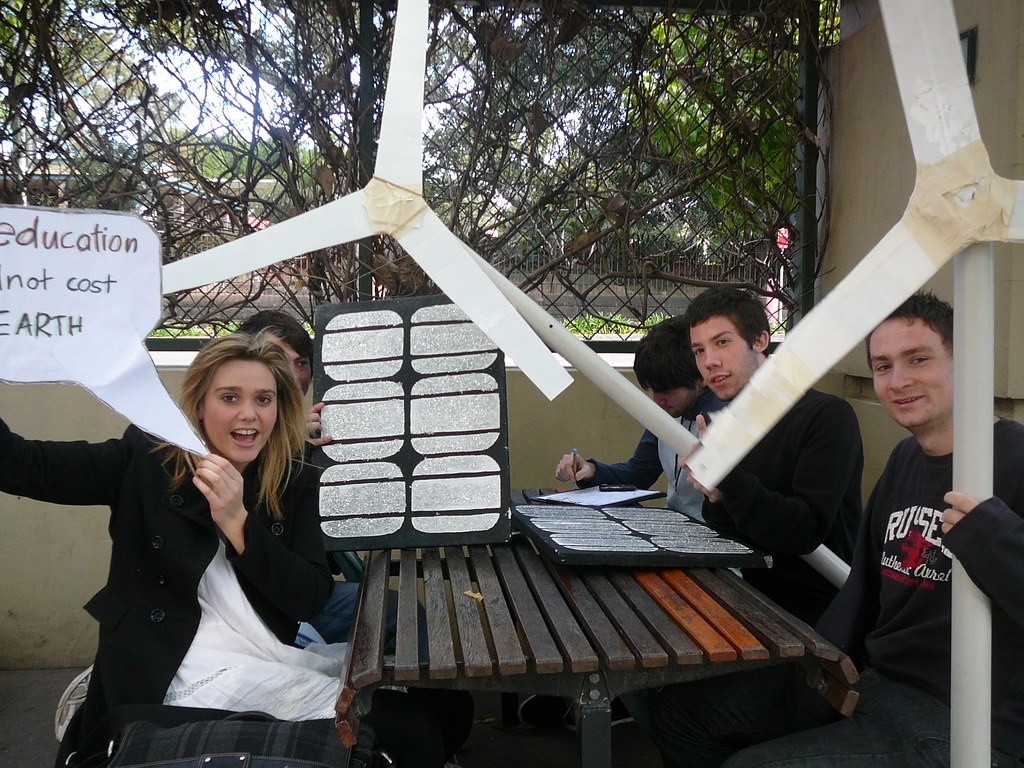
109;711;380;768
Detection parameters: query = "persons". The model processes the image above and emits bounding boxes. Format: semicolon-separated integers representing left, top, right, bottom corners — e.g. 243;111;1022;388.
555;312;728;528
647;288;1024;768
0;310;475;768
515;287;864;730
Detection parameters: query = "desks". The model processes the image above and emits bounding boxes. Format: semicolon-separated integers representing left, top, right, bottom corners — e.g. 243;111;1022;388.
335;486;861;768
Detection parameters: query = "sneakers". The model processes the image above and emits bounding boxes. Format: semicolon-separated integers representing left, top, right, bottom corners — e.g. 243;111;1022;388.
519;693;635;730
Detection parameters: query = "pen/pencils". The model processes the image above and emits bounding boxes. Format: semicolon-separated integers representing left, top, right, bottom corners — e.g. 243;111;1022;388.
572;447;578;491
